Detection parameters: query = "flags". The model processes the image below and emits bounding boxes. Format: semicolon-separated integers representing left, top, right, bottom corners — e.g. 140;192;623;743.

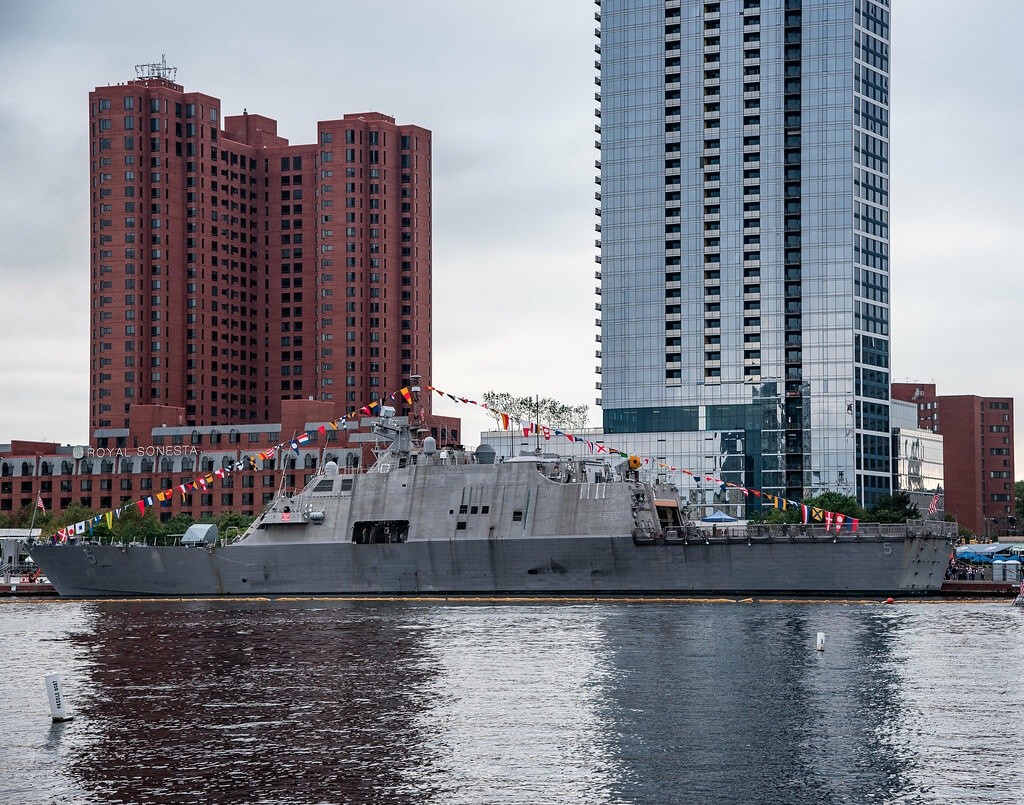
37;496;46;516
928;483;942;514
422;384;861;531
53;384;413;545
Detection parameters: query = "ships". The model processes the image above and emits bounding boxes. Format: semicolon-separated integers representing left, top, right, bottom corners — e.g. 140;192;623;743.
24;375;1024;605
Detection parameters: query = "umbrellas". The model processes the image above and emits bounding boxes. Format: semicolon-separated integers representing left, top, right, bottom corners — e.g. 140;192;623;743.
957;551;1024;565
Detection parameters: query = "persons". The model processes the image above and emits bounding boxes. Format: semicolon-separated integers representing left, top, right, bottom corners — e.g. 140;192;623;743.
707;531;711;538
28;572;36;583
781;522;788;537
946;565;987;580
790;522;807;538
712;524;717;538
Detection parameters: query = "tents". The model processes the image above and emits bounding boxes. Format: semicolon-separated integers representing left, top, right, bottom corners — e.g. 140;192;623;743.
700;509;740;539
955;544;1014;557
